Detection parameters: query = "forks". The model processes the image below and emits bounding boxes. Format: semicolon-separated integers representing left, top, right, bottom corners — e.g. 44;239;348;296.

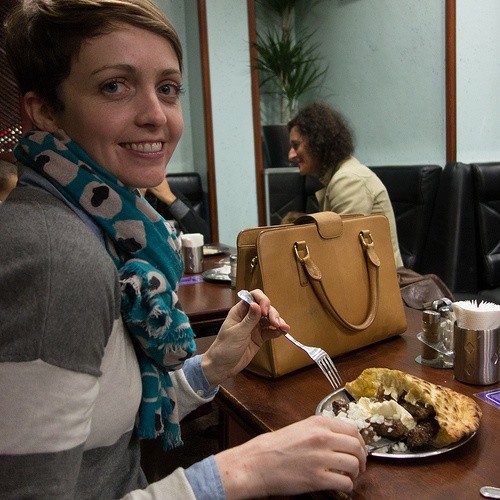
237;289;343;391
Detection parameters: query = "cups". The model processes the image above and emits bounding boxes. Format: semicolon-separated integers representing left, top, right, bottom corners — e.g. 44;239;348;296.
182;246;204;274
454;321;500;386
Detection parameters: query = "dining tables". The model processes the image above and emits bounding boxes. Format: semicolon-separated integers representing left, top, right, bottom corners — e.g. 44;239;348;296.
180;300;500;500
168;242;237;337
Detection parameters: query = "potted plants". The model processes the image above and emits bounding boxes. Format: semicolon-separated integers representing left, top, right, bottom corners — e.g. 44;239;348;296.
248;0;332;168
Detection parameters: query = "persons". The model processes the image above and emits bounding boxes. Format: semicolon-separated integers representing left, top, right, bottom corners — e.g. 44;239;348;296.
137;175;210;247
0;141;21;204
0;0;369;500
289;102;404;271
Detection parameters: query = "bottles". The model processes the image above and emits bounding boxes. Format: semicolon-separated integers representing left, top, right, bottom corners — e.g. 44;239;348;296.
439;306;453;352
230;254;237;289
423;310;440;360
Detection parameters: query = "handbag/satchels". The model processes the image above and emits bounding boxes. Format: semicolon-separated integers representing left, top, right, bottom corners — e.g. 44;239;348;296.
396;265;455;311
234;211;406;377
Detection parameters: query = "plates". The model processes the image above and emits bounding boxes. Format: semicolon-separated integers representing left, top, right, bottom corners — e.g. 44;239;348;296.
316;388;475;458
203;246;228;256
202;267;232;281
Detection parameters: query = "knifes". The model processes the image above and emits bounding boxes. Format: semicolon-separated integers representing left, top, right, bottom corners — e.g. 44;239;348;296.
365;437;398;455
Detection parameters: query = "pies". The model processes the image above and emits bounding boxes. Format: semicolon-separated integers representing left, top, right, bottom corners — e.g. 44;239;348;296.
343;367;482;448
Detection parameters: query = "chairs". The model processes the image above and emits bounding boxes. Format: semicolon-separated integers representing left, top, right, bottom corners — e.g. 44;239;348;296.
142;172;205;243
438;161;500;308
304;160;442;279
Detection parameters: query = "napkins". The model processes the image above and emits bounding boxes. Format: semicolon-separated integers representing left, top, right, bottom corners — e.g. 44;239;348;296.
450;299;500;385
